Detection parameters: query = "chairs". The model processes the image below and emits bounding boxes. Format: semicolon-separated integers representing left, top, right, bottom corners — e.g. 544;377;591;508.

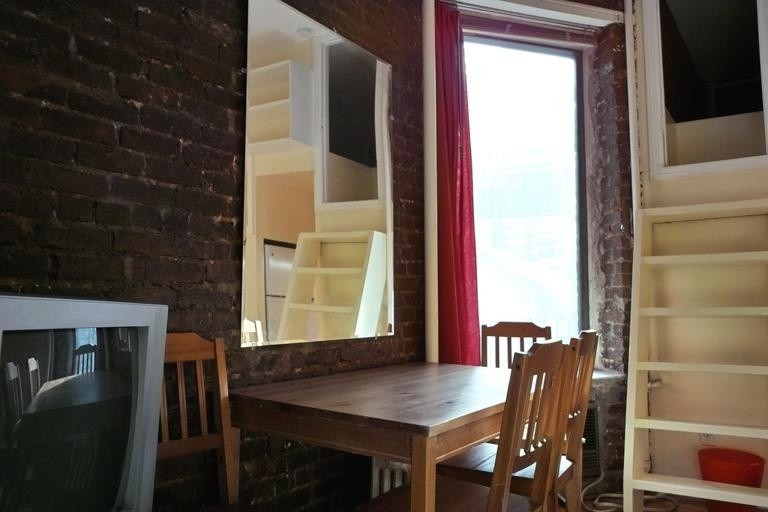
156;331;241;506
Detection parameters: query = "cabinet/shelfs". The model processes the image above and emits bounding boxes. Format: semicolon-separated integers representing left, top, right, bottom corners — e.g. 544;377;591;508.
249;59;313;177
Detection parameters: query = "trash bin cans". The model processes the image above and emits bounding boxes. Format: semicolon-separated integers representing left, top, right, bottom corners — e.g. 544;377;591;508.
699;448;764;512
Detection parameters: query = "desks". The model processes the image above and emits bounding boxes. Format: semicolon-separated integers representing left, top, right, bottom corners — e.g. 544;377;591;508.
221;360;625;511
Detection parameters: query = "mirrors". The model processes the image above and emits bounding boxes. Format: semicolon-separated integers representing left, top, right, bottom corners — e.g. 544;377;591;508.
238;1;396;348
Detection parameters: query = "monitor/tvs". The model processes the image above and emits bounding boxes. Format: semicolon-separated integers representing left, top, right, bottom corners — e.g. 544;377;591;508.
0;295;169;511
